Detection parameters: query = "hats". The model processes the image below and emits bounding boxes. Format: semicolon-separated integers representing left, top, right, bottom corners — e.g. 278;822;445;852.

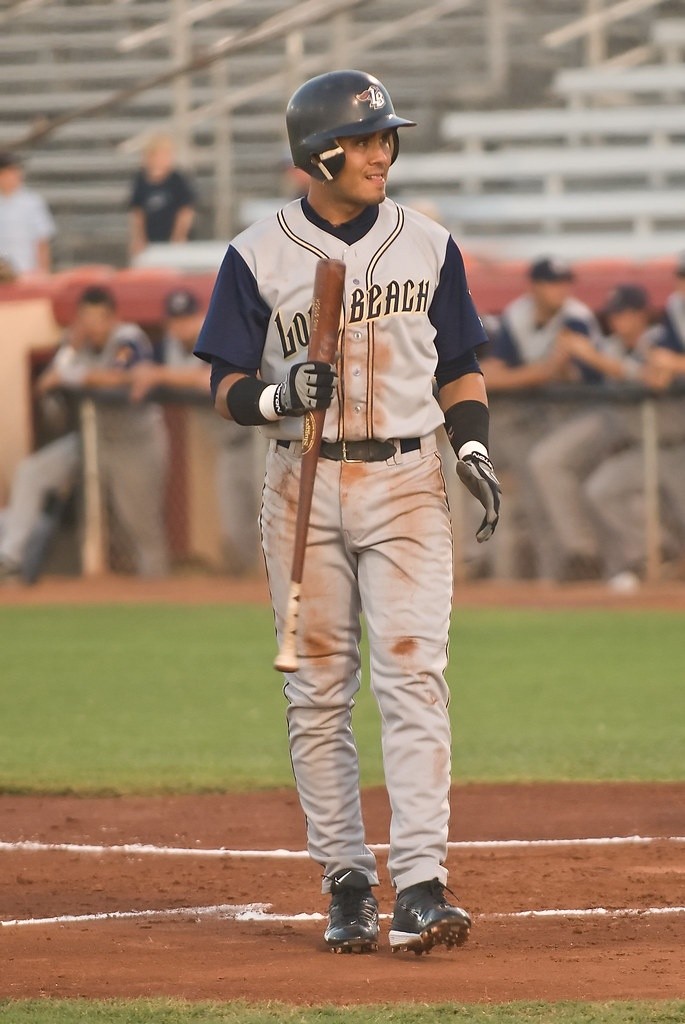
594;286;647;310
531;259;574;280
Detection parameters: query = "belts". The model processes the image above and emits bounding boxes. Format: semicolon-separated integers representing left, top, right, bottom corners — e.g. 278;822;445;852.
277;437;423;465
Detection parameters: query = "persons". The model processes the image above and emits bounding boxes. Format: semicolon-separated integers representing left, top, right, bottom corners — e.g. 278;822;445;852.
0;288;173;582
193;69;499;953
131;291;261;572
130;137;194;263
0;153;56;279
480;256;684;594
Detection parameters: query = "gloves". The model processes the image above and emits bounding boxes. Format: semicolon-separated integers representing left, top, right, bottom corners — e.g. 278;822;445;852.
273;352;339;416
456;451;501;542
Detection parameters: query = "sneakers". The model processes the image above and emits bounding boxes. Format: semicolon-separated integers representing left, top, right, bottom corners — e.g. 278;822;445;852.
320;869;380;953
388;878;472;955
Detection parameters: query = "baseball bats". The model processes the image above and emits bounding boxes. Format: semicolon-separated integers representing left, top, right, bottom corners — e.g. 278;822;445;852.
274;259;346;672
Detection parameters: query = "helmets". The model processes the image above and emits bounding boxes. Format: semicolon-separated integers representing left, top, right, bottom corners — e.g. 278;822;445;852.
286;70;417;181
